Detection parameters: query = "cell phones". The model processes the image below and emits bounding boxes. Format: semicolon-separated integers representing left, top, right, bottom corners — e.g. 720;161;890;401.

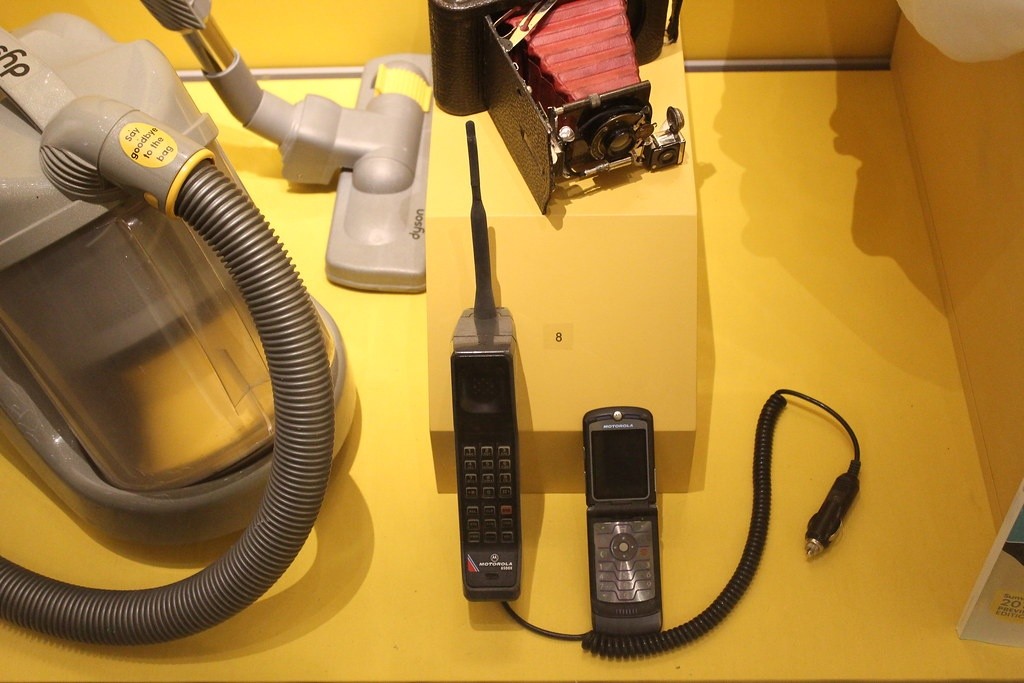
584;405;663;636
